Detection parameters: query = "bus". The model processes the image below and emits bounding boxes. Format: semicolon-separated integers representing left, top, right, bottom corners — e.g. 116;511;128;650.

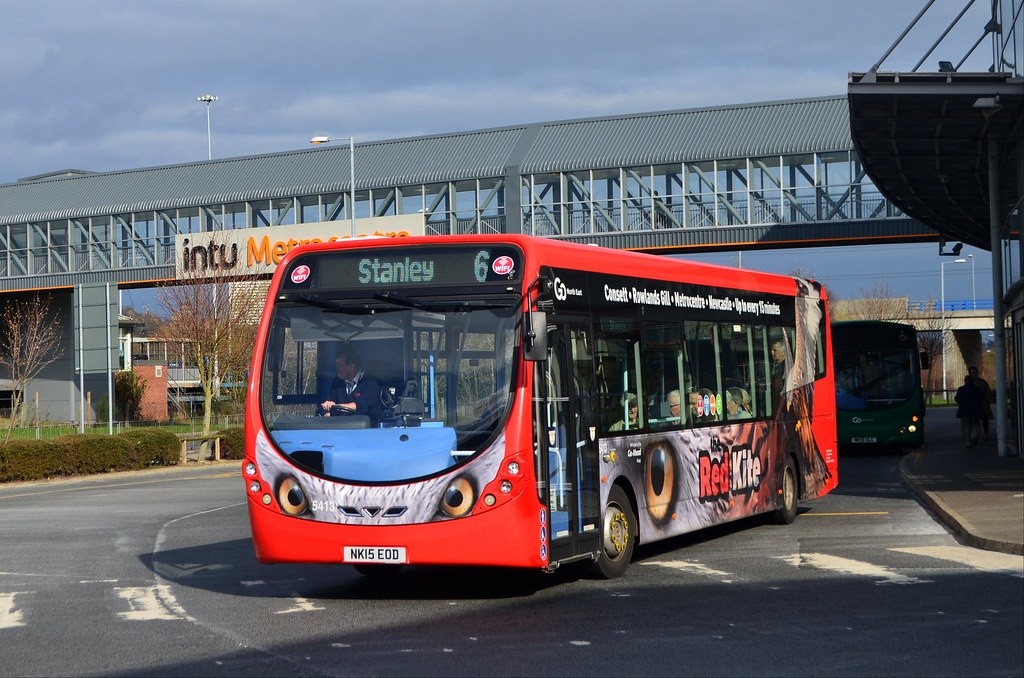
240;233;841;580
830;319;931;458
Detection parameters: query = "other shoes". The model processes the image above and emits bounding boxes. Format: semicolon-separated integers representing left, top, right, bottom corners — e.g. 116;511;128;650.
973;436;978;446
984;433;989;439
966;443;972;448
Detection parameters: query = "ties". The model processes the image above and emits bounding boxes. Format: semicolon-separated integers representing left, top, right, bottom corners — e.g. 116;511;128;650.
346;381;356;394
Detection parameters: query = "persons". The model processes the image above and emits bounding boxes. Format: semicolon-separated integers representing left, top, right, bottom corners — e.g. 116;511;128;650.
954;365;994;447
766;339;794;399
320;350;380;423
657;387;754;425
608;392;639;431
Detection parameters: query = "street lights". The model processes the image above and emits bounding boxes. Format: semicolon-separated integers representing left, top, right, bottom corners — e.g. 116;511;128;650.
196;94;221;161
310;136;357;238
967;254;977;311
940;259;967;400
